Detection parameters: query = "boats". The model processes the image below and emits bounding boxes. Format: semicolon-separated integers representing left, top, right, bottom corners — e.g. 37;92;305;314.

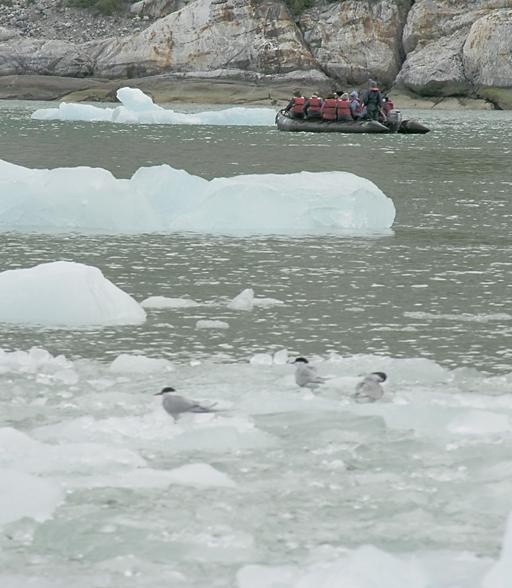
275;108;430;134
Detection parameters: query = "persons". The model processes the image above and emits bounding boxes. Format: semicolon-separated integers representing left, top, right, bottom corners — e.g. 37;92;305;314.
285;81;393;121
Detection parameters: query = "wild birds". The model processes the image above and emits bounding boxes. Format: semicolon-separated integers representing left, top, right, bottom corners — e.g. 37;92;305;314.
351;371;387;404
289;357;338;394
153;386;221;424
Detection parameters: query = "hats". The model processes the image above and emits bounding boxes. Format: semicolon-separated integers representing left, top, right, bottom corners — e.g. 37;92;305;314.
293;79;379;98
